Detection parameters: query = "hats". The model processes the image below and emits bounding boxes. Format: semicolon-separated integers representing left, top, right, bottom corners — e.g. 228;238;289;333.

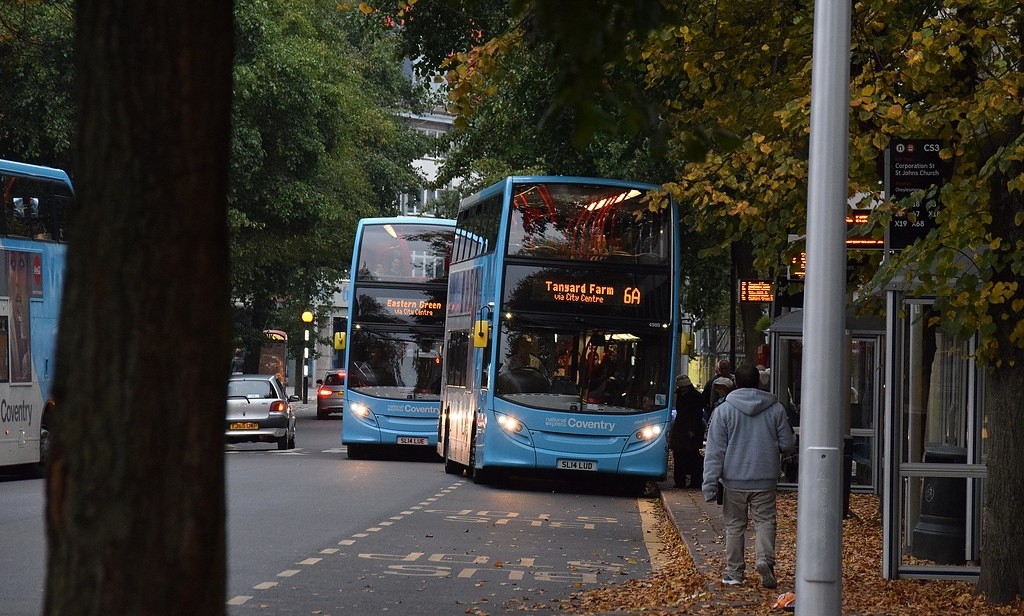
676;375;693;386
714;377;734;389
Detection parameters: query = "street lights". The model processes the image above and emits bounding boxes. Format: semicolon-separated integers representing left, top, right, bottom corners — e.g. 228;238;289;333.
301;307;314;404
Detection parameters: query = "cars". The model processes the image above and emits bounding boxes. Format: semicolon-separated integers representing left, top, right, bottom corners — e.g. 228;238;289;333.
225;373;301;450
316;369;345;420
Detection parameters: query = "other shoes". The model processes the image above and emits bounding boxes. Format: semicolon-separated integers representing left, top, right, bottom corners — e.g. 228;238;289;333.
691;479;703;487
674;479;687;488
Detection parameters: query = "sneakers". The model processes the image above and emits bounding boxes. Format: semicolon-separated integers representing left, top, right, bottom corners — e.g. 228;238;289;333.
756;562;778;588
722;571;744;585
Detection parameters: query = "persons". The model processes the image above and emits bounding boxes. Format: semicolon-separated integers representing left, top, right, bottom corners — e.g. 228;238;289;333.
669;374;703;491
497;334;552;387
759;371;770;391
701;364;797;589
588;352;599;368
702;361;736;417
357;348;386;386
709;377;734;408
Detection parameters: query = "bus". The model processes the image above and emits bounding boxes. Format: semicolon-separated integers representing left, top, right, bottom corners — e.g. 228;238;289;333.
334;218;488;459
436;175;694;497
0;159;75;479
